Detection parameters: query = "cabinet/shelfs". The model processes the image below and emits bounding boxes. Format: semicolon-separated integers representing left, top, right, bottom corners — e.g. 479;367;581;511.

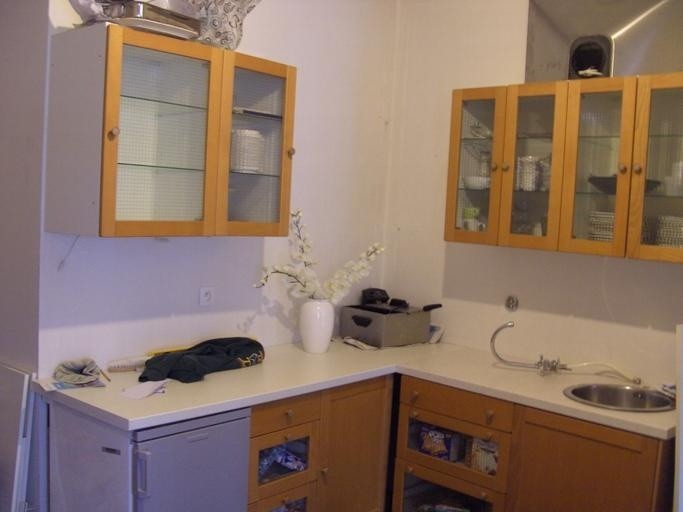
44;23;299;238
442;70;683;266
251;380;392;512
392;374;673;510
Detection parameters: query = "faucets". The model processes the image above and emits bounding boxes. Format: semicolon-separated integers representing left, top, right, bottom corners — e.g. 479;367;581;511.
489;319;571;375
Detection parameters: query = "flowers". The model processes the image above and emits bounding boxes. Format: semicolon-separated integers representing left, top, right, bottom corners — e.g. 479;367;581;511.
249;205;388;306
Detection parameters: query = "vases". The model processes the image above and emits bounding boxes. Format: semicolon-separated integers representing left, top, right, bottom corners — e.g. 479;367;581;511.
299;297;335;355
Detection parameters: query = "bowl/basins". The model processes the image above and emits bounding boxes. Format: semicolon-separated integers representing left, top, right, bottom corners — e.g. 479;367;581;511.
463;176;490;190
514;156;541;192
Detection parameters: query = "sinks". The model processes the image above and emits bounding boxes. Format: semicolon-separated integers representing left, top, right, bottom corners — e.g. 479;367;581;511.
563;381;676;413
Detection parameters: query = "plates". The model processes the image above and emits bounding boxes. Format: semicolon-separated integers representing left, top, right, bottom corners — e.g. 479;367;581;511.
588;211;683;249
589;176;660;195
231;129;264;174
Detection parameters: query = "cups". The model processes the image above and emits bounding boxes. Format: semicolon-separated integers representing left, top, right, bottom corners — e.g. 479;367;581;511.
465;220;486;234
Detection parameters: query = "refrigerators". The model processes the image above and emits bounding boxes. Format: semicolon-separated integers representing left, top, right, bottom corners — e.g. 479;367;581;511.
48;406;254;512
